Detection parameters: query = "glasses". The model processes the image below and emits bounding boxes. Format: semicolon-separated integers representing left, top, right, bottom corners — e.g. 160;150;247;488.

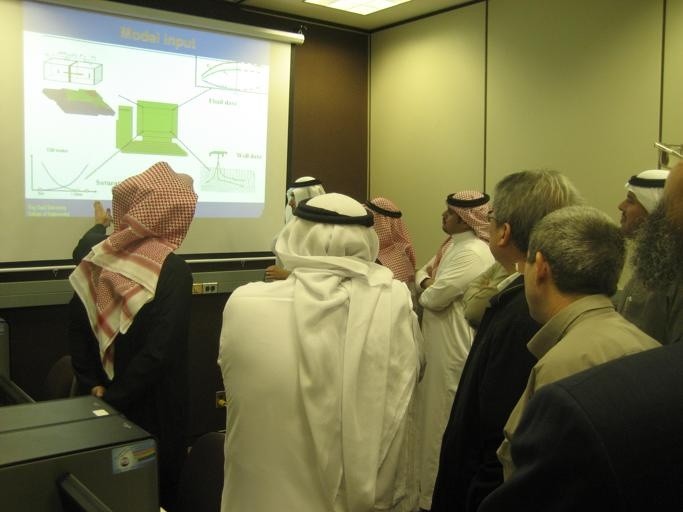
485;208;496;224
653;141;683;172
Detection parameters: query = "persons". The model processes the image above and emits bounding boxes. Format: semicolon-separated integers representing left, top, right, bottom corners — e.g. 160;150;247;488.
475;154;681;511
427;166;587;512
615;168;671;240
60;162;197;512
362;196;423;331
411;187;498;512
493;203;661;486
264;174;328;282
214;191;425;512
